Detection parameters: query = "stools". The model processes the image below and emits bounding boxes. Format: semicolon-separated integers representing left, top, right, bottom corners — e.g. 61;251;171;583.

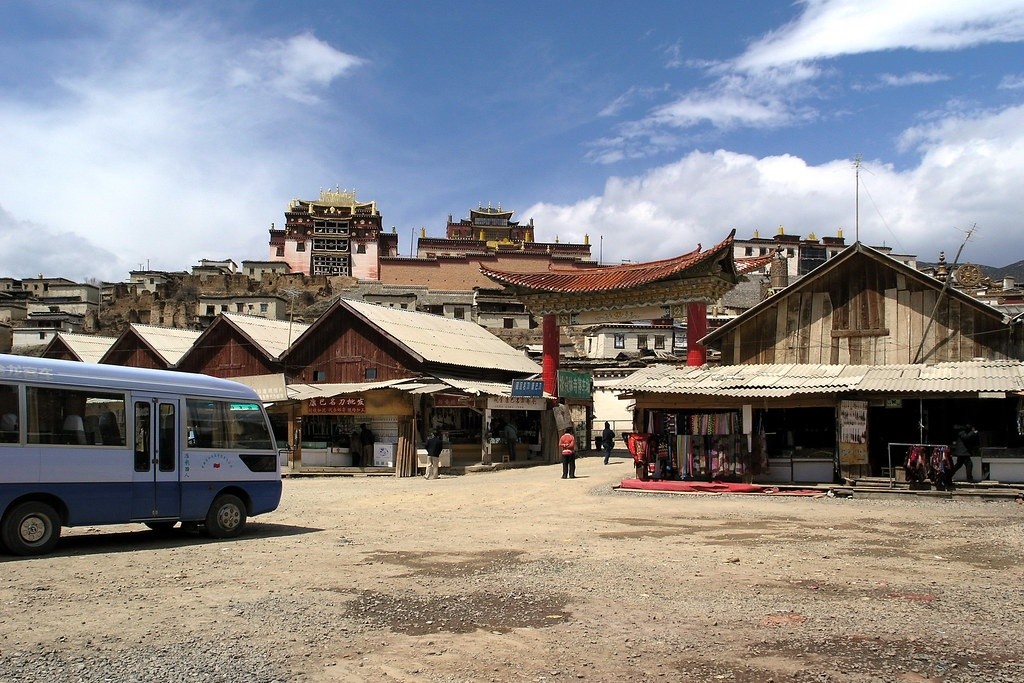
502;455;509;462
881;467;894;476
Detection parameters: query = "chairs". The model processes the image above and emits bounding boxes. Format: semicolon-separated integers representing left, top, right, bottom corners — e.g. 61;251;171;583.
0;411;176;457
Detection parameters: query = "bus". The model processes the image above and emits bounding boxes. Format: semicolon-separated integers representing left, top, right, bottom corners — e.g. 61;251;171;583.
0;353;282;556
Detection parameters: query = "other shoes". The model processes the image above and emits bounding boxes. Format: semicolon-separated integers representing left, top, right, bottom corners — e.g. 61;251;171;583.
968;480;978;483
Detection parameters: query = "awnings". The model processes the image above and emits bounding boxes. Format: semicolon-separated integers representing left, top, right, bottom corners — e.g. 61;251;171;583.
610;364;1024;398
433;371;557;400
392;382;452;394
287;379;414;399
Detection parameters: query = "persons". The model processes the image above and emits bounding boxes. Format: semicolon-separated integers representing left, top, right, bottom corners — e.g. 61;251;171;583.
423;429;443;479
559;429;576;478
952;425;976;483
603;421;615;465
505;422;517;460
351;424;374;466
